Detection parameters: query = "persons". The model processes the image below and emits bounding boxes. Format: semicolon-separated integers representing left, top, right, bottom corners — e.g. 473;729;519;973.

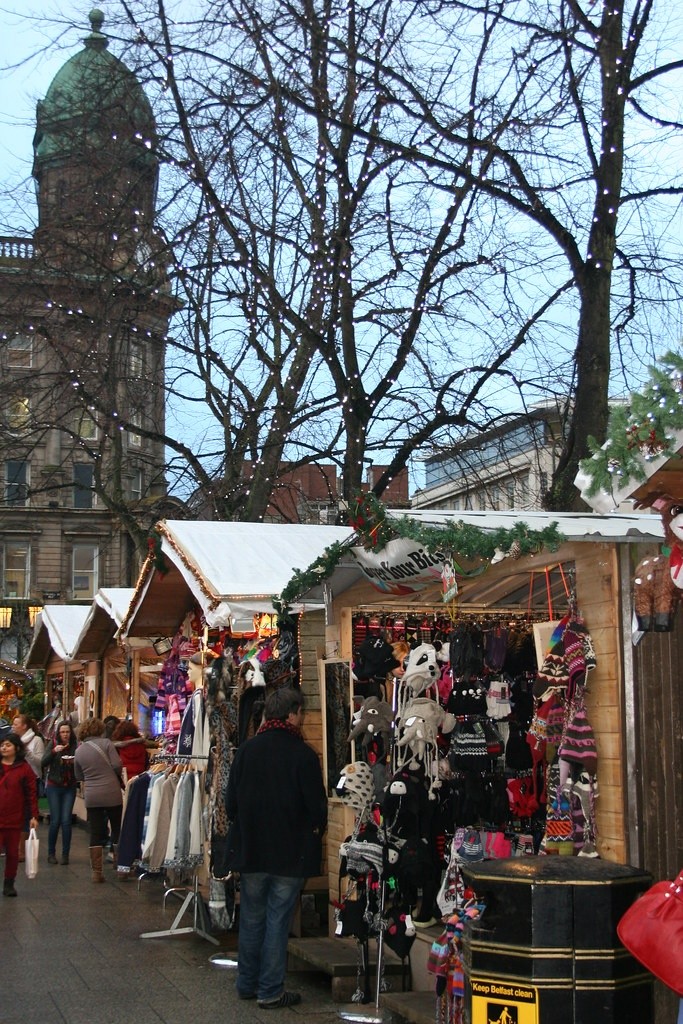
176;652;203;765
386;639;413;701
73;719;124;883
0;734;39;899
41;721;80;866
111;722;147;792
223;688;327;1009
10;713;46;802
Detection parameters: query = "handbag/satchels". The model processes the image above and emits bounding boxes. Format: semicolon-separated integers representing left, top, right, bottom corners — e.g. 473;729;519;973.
118;776;125;791
25;827;39;879
615;869;683;994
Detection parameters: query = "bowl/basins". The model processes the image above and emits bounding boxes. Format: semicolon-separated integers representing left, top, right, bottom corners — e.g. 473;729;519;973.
62;757;74;764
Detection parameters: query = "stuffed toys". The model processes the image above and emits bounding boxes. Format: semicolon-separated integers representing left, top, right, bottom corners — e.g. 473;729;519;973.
402;639;442;697
397;700;456;764
347;696;393;747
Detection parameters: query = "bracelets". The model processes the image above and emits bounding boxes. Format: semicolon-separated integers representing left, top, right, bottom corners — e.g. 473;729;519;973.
31;817;37;819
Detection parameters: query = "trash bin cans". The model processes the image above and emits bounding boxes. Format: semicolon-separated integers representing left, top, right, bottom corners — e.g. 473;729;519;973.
459;855;657;1024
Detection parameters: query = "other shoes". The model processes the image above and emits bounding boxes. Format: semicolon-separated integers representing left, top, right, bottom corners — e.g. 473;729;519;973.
59;857;69;865
259;991;301;1008
107;853;113;860
48;857;58;864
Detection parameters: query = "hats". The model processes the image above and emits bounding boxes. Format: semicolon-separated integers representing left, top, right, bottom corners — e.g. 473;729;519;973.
334;606;601;1023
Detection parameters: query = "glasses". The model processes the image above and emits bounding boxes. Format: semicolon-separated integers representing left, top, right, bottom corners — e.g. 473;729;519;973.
59;730;70;734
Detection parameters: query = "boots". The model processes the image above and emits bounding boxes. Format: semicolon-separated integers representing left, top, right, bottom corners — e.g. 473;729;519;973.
3;879;17;896
113;843;119;867
88;845;105;882
18;832;26;862
